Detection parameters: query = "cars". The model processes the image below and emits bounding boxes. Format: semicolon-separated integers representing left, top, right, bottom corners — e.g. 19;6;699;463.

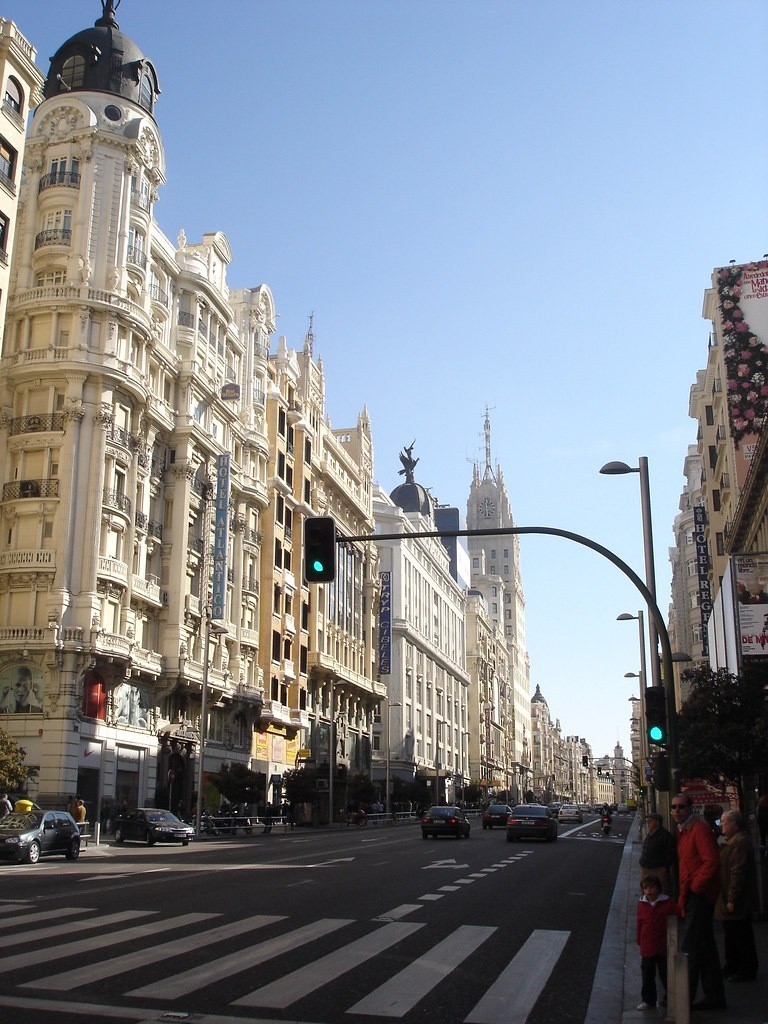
527;801;631;819
481;805;514;830
506;804;560;843
420;805;471;839
558;804;584;824
112;807;195;847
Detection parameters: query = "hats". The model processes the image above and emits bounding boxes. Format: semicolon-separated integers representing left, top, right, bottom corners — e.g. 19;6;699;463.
646;813;663;819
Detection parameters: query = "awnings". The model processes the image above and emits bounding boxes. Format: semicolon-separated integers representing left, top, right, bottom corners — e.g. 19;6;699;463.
393;769;416;785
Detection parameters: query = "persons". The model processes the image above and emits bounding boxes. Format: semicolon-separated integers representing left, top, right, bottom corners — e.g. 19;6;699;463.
703;802;724;848
0;665;44;713
339;800;385;824
599;801;612;828
736;580;761;604
177;800;296;832
672;793;730;1011
636;875;679;1010
0;793;13;820
391;798;514;823
712;807;762;982
64;793;87;835
641;811;674;899
755;794;768;857
101;797;131;835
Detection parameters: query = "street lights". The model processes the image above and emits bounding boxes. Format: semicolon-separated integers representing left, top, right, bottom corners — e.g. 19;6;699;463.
384;695;402;823
615;609;654;814
630;716;648;816
598;455;695;687
435;719;448;806
195;618;230;834
327;679;348;826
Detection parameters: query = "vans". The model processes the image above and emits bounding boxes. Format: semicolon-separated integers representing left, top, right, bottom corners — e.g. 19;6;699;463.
0;792;41;810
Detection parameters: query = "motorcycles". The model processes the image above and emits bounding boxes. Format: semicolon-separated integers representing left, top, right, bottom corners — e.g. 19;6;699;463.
598;810;616;835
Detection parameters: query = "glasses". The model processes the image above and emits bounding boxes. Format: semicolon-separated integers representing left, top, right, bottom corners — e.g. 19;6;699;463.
671;804;685;809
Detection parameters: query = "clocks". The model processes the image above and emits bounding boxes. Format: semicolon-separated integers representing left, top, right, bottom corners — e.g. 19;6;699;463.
479;500;495;516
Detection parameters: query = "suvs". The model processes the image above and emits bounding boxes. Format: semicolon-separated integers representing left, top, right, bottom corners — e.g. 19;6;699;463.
0;809;81;865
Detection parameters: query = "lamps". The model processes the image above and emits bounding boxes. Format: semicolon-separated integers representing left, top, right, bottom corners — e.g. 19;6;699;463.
730;259;736;265
763;254;768;261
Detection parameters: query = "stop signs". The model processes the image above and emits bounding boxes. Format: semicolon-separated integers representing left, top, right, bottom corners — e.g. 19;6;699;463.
168;769;175;784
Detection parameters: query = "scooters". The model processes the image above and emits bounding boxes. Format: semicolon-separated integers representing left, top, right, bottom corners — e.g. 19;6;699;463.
175;803;254;837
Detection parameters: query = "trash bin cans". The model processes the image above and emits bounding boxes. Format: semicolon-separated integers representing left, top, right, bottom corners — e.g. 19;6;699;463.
15;800;33;812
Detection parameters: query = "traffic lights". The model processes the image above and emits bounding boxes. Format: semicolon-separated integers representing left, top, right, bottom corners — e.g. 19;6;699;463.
643;685;668;744
597;767;602;775
621;787;624;792
303;516;337;585
612;779;615;785
606;772;609;779
582;755;588;767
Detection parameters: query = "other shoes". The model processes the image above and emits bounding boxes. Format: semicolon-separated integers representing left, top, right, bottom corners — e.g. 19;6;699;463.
728;972;757;983
659;994;668;1007
692;1000;727;1012
637;1002;652;1010
